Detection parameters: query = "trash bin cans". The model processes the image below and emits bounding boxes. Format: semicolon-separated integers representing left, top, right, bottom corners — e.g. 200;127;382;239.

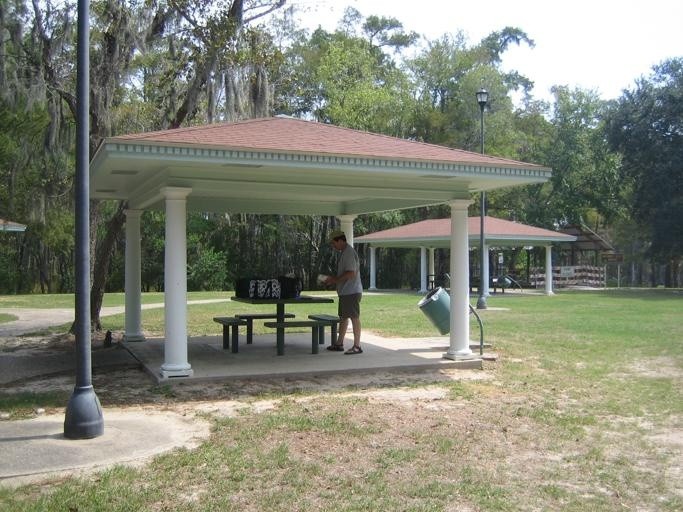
417;287;450;335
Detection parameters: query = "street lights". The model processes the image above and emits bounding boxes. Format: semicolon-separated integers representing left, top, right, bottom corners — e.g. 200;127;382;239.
475;88;489;309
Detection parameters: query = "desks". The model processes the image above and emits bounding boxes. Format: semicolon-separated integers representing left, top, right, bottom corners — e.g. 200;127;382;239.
232;295;333;355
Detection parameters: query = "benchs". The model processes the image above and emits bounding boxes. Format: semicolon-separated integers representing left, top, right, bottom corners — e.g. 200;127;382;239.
212;315;342;355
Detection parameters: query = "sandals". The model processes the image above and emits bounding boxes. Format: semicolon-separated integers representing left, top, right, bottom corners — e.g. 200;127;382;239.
326;344;343;351
343;345;363;354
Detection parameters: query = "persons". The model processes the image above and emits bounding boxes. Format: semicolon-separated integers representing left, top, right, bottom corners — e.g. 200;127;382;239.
317;229;365;355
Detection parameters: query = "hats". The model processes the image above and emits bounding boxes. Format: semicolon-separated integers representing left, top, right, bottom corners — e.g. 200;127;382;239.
328;230;344;243
